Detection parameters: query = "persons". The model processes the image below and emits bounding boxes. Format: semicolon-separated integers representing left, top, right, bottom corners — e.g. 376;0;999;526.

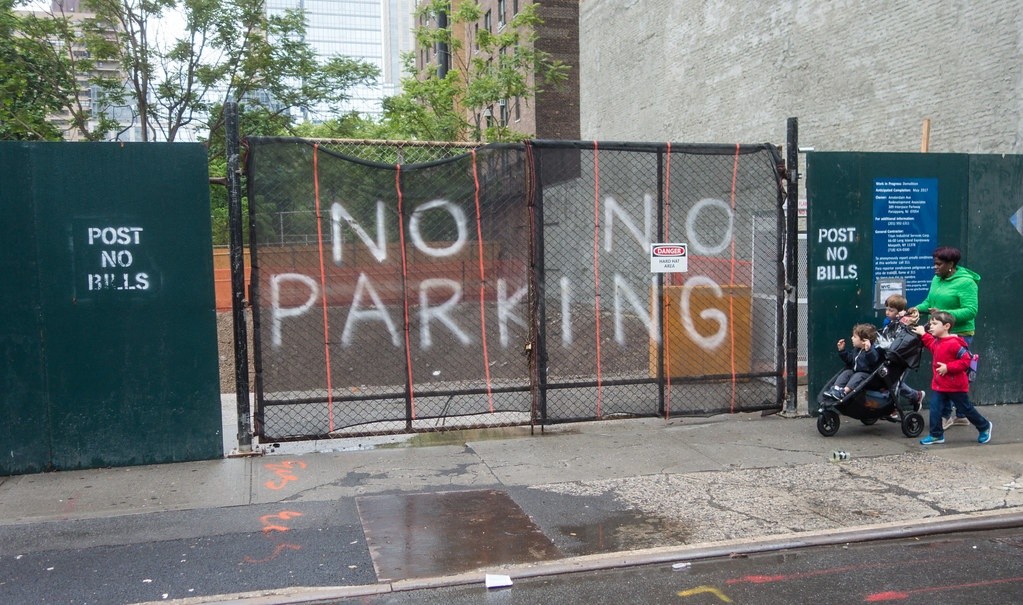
907;247;982;430
823;322;879;400
911;311;992;444
880;294;925;420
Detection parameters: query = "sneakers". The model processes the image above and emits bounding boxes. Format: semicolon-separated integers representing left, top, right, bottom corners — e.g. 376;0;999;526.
881;410;898;420
978;422;992;444
941;417;953;430
823;386;838;397
951;416;969;425
833;388;847;401
914;391;925;412
920;435;944;444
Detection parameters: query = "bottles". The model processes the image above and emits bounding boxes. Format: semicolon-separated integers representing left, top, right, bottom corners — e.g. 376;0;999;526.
968;354;979;382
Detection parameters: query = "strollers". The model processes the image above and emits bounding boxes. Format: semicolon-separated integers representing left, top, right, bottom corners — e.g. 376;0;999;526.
814;308;928;440
